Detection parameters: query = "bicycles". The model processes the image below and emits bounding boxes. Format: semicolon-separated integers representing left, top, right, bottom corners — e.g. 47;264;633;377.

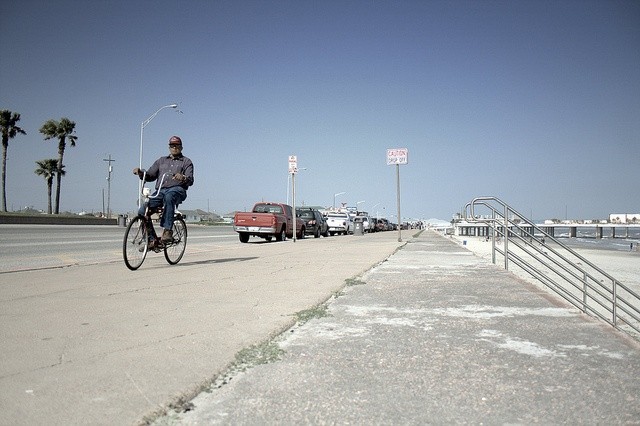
123;168;188;270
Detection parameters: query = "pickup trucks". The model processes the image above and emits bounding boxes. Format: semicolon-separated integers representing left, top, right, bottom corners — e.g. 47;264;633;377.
325;211;353;236
233;201;306;243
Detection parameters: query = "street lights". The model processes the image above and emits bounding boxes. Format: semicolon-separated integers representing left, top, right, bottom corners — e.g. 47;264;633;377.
138;104;178;213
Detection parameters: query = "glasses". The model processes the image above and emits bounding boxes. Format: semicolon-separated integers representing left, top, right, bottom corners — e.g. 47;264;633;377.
170;144;181;148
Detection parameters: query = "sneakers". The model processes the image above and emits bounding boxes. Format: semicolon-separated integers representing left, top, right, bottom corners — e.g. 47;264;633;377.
139;240;160;252
161;230;174;241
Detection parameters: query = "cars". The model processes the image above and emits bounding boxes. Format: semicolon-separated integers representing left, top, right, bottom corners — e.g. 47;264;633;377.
296;209;330;238
353;215;375;232
376;217;423;230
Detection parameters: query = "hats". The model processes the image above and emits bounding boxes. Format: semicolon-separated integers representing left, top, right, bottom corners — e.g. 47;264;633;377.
169;136;182;144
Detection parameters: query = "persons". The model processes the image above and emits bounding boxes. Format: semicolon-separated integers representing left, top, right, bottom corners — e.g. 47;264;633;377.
132;135;194;253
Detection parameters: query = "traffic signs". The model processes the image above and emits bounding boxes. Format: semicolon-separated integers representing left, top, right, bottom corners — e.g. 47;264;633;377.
386;147;408;165
